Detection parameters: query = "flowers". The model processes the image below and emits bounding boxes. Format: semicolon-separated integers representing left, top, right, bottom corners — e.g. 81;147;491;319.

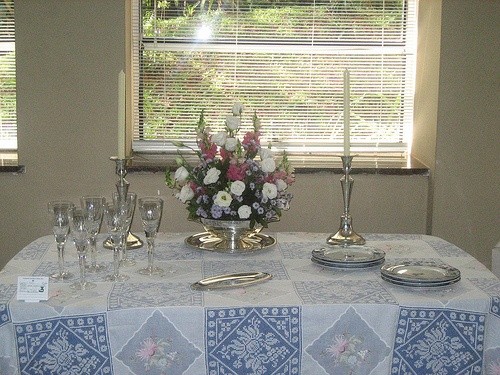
164;102;295;228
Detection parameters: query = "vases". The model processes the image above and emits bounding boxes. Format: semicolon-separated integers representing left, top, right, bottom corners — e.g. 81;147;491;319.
201;217;265;250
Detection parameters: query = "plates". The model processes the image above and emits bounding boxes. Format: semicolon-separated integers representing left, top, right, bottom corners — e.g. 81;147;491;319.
311;242;387;270
379;259;461;290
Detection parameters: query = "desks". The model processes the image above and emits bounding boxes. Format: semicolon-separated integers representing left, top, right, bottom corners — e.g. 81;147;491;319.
0;232;500;375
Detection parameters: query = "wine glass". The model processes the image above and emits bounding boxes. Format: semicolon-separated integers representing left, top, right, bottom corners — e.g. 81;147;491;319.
105;192;164;281
46;200;76;280
66;207;97;291
79;196;105;270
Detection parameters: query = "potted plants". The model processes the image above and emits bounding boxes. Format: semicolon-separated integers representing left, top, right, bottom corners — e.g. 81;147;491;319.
117;70;126;159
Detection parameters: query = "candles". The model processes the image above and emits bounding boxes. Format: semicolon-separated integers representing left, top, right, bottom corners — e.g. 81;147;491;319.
344;68;350;156
